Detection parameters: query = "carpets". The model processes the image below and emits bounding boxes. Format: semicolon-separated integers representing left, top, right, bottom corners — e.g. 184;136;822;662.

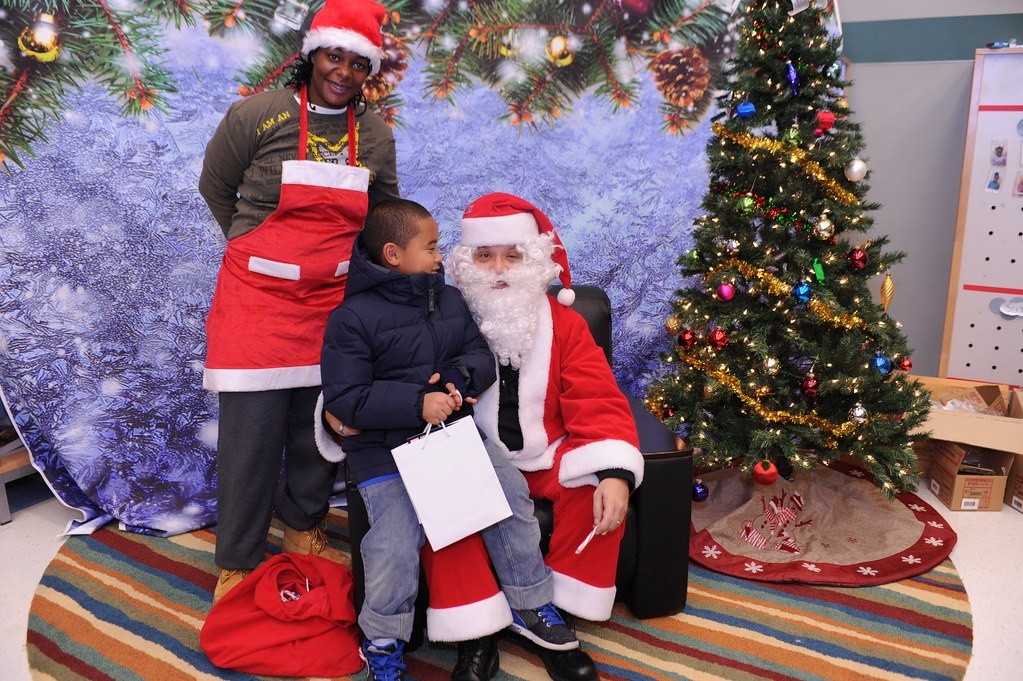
26;520;978;681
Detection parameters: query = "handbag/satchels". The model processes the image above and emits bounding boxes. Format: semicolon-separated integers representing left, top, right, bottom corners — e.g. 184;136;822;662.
391;388;514;552
197;552;365;677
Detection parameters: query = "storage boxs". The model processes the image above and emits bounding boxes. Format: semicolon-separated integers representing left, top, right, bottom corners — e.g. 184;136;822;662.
905;380;1023;512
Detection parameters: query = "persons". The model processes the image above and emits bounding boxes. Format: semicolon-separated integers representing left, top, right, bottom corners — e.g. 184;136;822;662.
196;2;402;603
312;193;645;681
319;199;579;681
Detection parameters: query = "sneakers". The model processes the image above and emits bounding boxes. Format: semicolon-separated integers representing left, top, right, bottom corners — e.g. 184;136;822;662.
282;521;352;568
508;600;580;651
211;567;254;606
357;637;407;681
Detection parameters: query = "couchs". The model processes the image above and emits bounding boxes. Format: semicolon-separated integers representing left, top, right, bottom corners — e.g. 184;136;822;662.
347;283;690;617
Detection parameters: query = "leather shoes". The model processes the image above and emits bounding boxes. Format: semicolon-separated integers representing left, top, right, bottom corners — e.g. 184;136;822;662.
526;639;600;681
451;637;500;681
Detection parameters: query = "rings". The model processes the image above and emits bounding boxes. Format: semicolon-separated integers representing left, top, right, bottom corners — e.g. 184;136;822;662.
616;519;621;524
338;424;346;434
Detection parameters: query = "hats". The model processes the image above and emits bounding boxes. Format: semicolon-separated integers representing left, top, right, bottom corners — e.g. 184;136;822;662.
461;192;575;307
301;0;388;78
